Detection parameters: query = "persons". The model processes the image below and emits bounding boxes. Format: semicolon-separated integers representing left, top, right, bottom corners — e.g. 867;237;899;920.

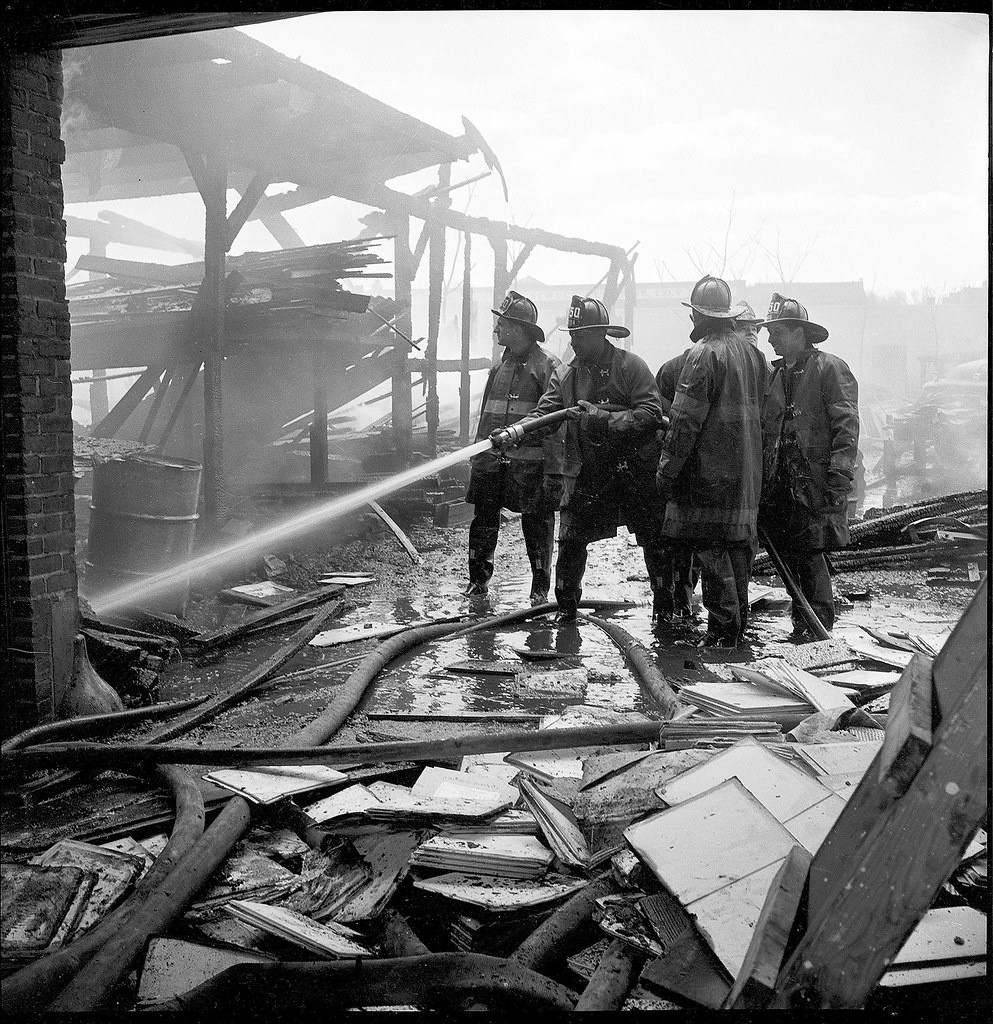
756;292;860;645
653;275;773;650
461;291;565;609
490;293;664;626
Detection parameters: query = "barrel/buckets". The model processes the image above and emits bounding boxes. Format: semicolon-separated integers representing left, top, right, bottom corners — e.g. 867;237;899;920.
84;452;203;616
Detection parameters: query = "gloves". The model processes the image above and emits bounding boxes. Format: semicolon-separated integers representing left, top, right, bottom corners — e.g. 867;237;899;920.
655;472;676;500
489;426;525;453
565;399;612;429
815;470;853;514
542;475;564;494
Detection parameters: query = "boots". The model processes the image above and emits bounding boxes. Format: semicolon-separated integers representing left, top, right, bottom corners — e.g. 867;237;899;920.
650;582;832;647
528;568;551;607
459;558;496;598
539;583;584;630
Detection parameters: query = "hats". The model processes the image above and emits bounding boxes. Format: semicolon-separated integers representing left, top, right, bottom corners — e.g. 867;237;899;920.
680;273;746;318
558;295;631;339
756;292;830;344
491;291;545;343
734;300;765;335
